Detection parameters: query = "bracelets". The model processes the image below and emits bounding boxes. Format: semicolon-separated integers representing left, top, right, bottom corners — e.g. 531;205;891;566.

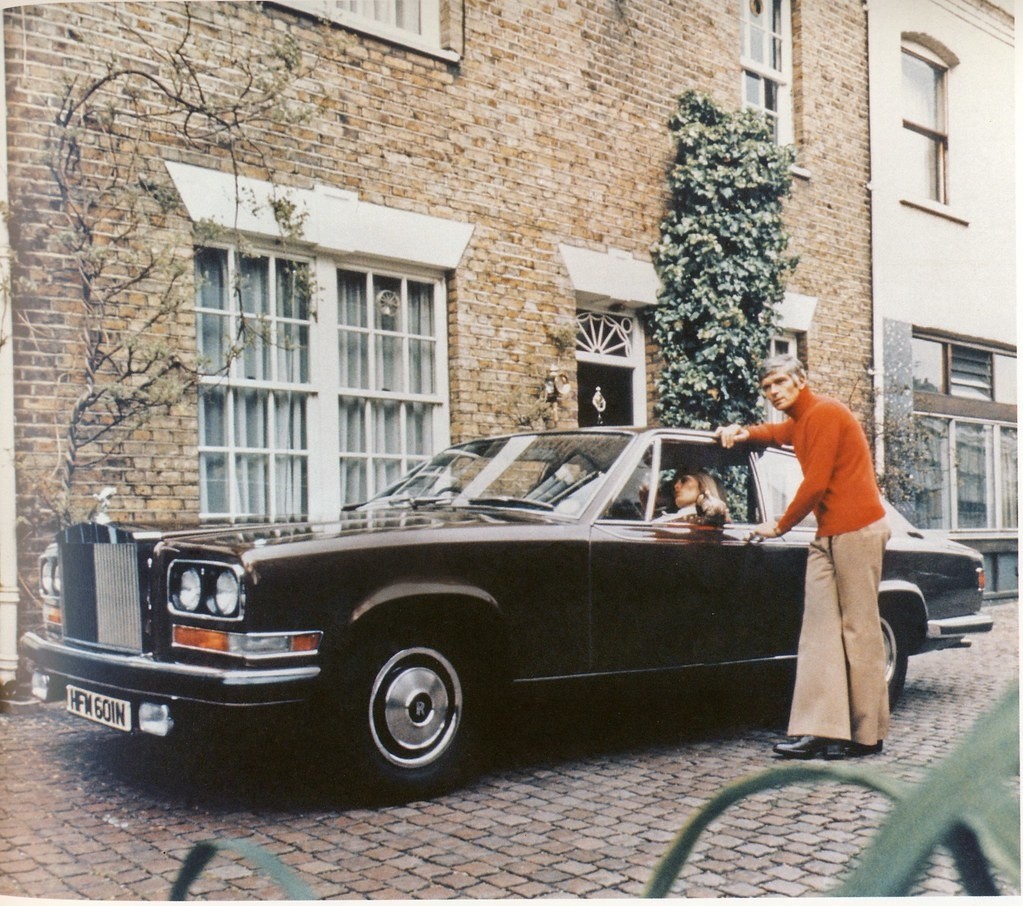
773;526;781;538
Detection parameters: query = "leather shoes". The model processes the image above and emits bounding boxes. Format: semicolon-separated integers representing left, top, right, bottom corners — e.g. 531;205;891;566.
815;735;882;762
773;732;846;758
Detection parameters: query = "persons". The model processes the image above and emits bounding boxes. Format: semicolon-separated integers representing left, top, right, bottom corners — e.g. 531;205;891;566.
716;356;890;759
640;468;733;523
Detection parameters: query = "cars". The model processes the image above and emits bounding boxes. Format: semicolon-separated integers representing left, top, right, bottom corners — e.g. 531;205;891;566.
17;425;996;811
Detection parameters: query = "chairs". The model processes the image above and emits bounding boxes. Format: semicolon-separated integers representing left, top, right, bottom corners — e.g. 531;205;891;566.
662;466;721;517
